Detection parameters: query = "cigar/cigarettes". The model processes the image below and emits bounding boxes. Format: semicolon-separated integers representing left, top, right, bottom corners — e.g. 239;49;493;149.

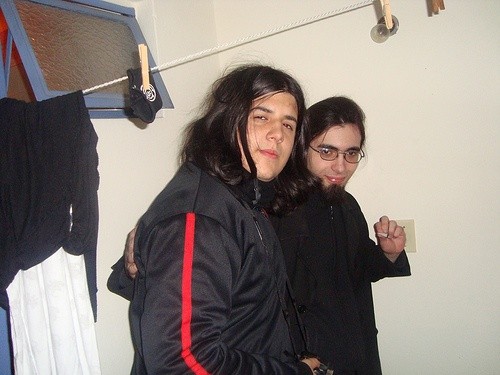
378;233;390;238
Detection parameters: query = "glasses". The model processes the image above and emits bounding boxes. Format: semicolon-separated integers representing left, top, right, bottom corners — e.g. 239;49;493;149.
308;143;365;163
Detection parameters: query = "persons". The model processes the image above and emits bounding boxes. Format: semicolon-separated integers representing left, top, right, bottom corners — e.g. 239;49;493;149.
107;96;412;375
128;65;323;375
